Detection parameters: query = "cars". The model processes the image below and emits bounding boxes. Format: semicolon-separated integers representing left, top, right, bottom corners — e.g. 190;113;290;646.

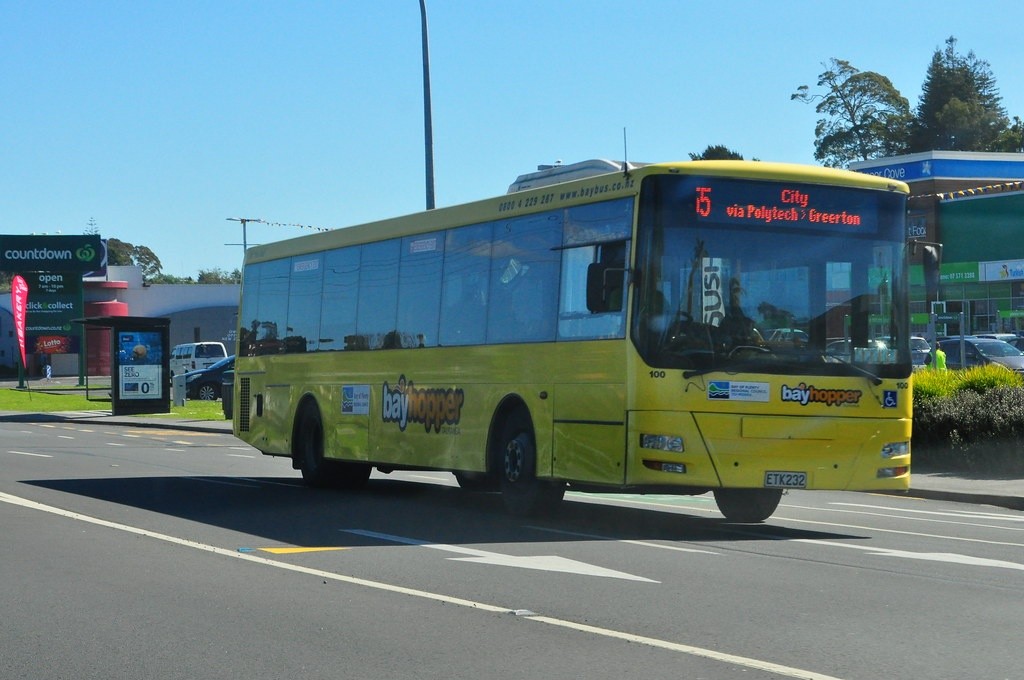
765;325;1024;382
184;354;236;401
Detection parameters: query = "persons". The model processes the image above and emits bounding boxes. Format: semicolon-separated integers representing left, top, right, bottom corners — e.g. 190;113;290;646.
709;277;756;352
924;342;947;372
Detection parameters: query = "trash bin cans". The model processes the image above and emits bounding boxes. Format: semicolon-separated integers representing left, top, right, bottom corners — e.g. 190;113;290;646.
222;370;234;420
173;374;186;406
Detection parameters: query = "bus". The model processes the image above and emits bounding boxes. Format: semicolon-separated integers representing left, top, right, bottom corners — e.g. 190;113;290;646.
228;158;944;526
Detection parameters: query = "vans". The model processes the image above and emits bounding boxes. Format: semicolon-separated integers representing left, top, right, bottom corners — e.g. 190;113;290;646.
170;341;228;385
